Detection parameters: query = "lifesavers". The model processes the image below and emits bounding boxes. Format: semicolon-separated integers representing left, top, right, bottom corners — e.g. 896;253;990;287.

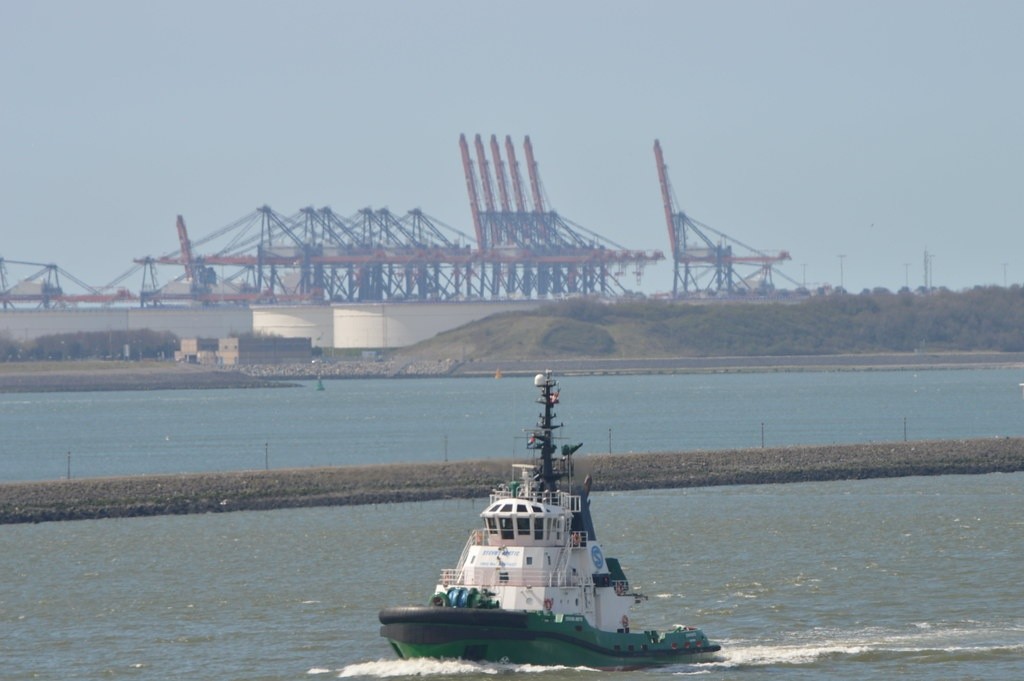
545;599;552;610
574;534;580;542
429;592;450;607
618;584;623;592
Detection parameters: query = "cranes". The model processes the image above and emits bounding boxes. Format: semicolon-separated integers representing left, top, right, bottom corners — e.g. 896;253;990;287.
654;139;795;301
2;131;668;311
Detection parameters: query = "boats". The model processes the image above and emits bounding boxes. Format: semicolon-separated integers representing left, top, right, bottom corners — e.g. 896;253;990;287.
377;364;731;675
